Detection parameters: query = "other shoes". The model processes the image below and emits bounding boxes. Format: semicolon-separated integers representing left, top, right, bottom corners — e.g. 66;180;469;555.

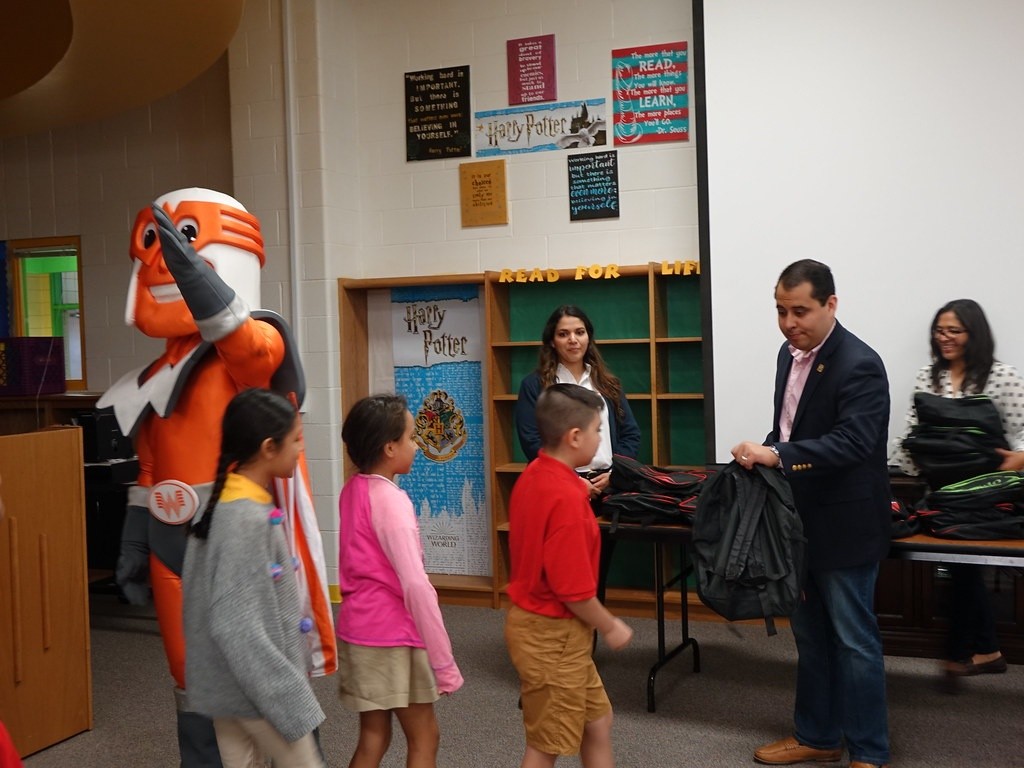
958;655;1007;676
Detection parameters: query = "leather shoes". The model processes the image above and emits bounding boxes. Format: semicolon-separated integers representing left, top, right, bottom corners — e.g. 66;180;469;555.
753;736;842;764
849;760;889;768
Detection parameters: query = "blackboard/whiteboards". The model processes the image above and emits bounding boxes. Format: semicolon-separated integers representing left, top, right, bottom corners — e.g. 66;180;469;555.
567;150;619;220
404;64;472;162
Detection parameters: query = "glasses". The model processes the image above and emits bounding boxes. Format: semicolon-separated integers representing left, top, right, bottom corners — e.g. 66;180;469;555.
933;326;967;338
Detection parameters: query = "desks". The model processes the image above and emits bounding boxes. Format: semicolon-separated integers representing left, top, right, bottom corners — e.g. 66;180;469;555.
0;393;116;442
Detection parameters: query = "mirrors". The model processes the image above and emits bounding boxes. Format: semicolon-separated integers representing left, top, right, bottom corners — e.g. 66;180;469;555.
7;236;87;390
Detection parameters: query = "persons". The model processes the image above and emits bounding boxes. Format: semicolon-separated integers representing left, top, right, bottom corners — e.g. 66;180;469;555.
504;383;635;768
730;259;893;768
181;384;327;768
514;306;642;658
336;394;465;768
886;299;1024;674
97;186;309;768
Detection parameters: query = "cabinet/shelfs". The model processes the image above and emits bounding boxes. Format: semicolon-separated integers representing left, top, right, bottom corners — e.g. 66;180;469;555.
866;477;1024;666
483;262;702;610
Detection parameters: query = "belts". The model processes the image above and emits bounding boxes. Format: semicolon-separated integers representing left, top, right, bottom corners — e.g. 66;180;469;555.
577;465;611;481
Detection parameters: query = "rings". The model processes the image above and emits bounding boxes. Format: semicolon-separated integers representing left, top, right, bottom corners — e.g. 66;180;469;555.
741;456;747;461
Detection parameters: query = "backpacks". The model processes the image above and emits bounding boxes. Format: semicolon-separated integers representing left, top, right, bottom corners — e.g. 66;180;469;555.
691;459;809;621
601;391;1024;540
